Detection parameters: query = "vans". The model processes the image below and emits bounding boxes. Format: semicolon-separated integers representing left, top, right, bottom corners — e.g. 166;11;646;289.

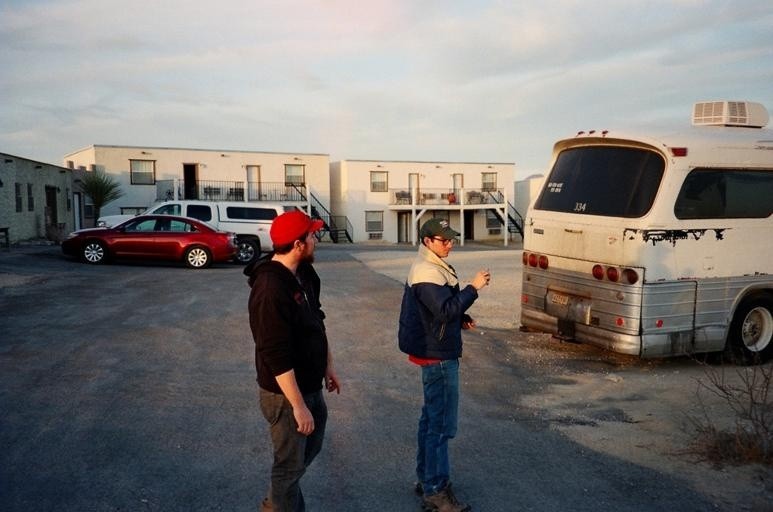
97;201;286;264
520;100;773;367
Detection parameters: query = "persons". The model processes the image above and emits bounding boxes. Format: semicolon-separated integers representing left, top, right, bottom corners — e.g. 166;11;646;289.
246;211;340;512
399;214;490;512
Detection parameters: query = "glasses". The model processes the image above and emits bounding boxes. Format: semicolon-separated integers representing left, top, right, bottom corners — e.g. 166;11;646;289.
432;237;452;245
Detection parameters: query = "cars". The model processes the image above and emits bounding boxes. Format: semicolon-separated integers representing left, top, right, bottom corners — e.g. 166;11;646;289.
62;215;239;268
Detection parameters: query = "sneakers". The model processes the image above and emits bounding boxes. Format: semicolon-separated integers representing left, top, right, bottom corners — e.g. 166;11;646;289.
416;481;471;512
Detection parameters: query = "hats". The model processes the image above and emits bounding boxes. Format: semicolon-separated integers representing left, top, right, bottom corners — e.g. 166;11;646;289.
420;218;460;239
270;211;324;247
